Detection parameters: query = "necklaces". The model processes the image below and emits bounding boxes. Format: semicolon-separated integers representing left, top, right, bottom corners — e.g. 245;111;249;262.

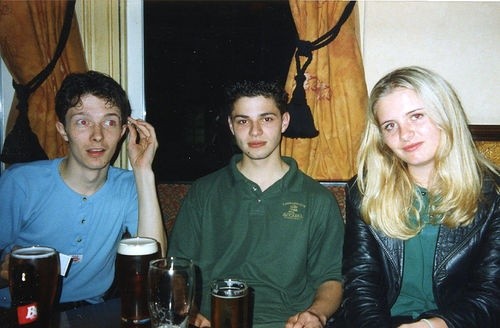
421;192;426;196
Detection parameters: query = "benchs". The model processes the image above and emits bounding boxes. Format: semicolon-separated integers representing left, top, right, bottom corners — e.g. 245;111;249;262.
155;180;350;243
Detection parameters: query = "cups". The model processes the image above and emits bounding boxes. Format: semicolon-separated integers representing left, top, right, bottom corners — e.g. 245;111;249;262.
7;244;61;328
114;236;162;328
209;276;251;328
149;257;197;328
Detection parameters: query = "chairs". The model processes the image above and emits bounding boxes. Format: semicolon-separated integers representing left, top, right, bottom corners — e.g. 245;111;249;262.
467;124;500;175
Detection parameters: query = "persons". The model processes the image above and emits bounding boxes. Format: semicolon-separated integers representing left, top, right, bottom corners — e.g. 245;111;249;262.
341;66;500;328
167;80;346;328
0;70;168;313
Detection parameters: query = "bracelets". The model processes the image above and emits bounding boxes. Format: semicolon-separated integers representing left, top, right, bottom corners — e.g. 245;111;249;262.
421;318;433;328
